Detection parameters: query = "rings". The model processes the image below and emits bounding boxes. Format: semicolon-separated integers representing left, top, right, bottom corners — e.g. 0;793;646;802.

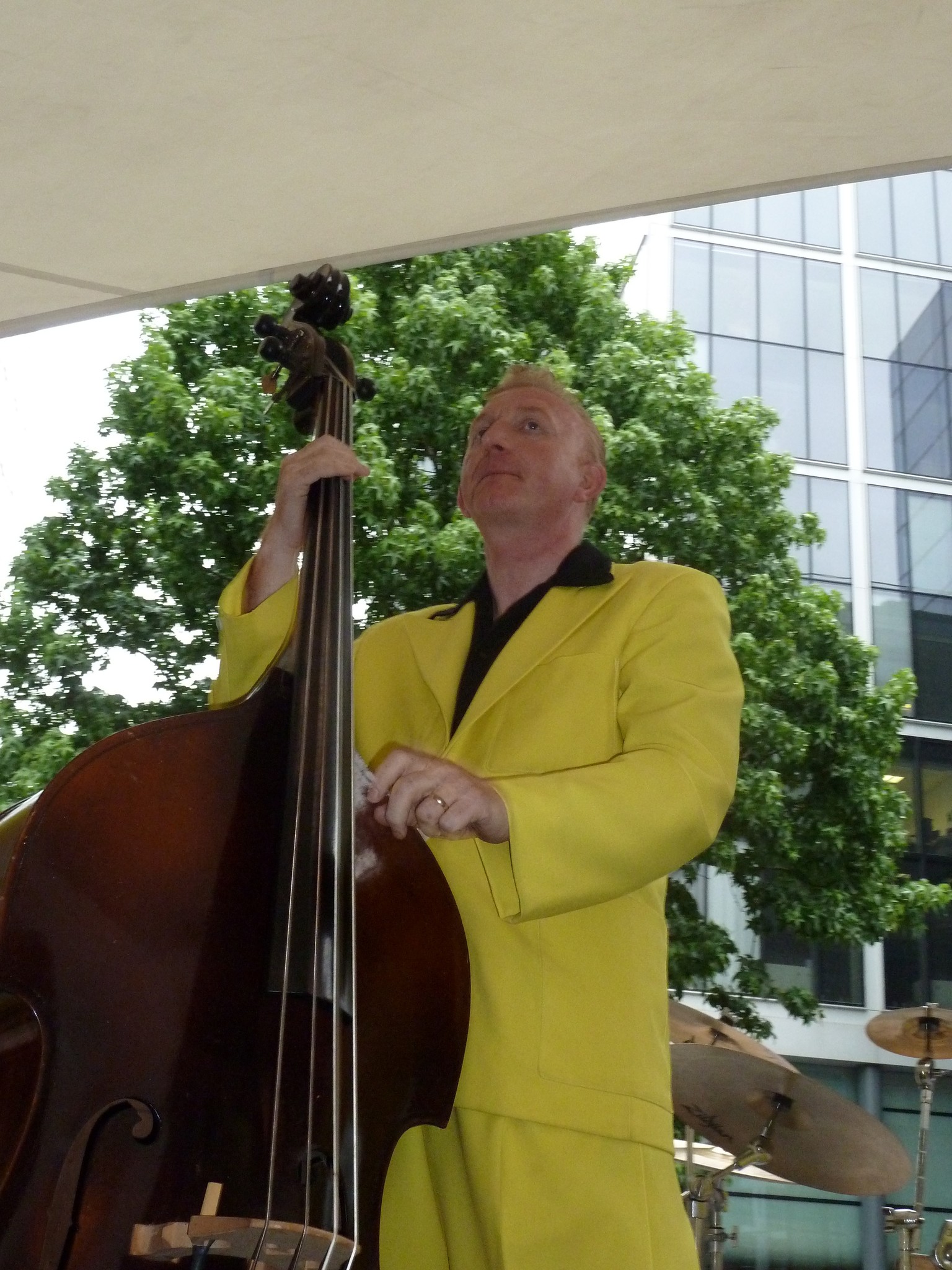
429;792;449;812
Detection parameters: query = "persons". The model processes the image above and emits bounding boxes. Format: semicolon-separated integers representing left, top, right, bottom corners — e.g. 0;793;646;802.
205;361;746;1269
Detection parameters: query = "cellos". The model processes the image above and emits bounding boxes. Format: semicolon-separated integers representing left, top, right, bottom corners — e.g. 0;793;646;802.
0;261;473;1269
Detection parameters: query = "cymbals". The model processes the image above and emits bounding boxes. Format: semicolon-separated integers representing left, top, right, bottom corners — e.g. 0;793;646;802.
864;1007;950;1058
668;1044;913;1198
668;998;803;1077
670;1137;796;1188
